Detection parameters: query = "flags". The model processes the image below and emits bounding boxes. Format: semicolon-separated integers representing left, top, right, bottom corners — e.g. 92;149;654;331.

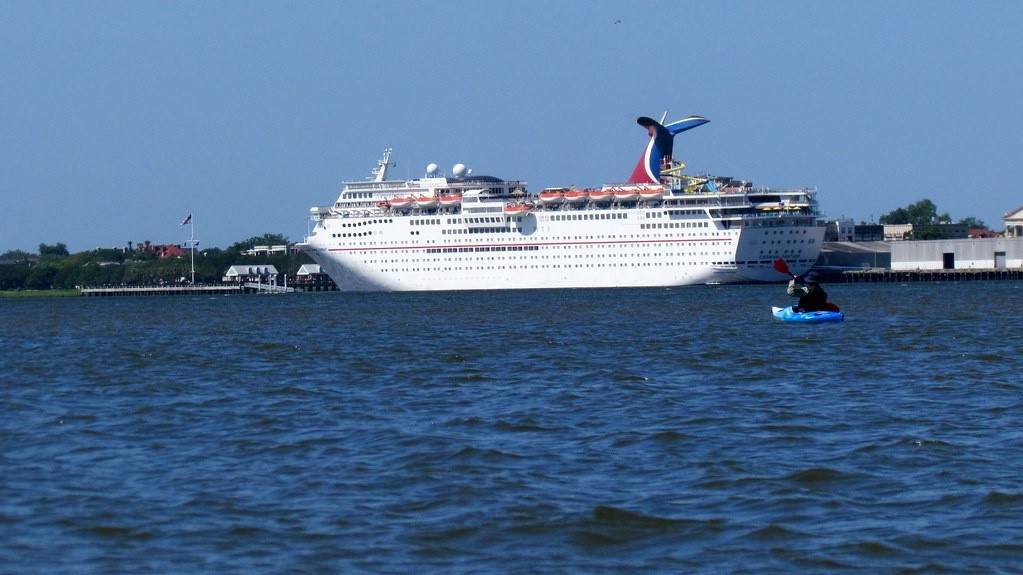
180;214;193;227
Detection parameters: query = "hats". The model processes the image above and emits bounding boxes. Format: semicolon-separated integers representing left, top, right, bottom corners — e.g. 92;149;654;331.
804;271;824;283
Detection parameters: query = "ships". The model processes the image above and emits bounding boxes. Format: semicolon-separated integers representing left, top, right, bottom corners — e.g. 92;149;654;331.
292;150;830;291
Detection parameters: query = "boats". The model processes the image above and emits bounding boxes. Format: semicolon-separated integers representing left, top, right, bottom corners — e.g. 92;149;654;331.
386;192;460;211
770;306;845;327
505;184;664;217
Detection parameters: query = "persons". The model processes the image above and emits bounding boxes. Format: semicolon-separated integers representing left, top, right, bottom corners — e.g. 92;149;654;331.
787;271;829;313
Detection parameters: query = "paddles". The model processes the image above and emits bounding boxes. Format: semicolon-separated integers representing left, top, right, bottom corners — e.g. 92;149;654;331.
774;258;840;312
